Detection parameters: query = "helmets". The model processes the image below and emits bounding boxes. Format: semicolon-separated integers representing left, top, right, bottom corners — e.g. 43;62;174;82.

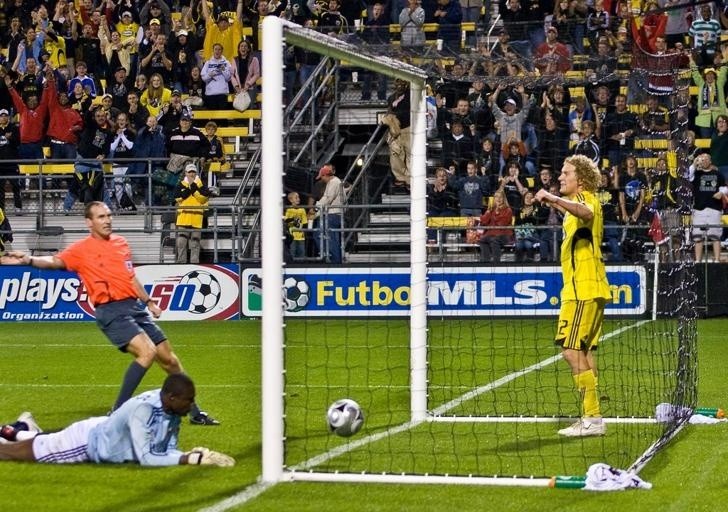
394;78;407;85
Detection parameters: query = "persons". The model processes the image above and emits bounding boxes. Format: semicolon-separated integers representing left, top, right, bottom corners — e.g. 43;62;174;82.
0;372;235;469
1;0;728;265
0;201;221;426
530;155;611;437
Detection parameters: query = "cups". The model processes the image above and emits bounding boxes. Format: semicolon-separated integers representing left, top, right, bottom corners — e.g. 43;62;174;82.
353;19;360;32
619;133;626;145
306;218;314;228
352;71;358;83
436;38;444;50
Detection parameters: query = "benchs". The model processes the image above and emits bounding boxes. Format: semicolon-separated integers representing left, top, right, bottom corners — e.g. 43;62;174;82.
344;21;691;262
513;18;514;20
18;77;262;216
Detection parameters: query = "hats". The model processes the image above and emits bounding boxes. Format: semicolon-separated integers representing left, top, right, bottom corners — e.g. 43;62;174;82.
625;153;637;162
547;27;558;34
115;66;126;73
498;29;507;35
179;113;191;121
171;89;181;98
596;36;610;46
149;18;161;26
176;29;189;37
102;93;112;101
185;164;198;173
150;2;162;9
315;165;336;180
704;67;717;75
121;11;132;19
0;109;9;116
503;98;517;107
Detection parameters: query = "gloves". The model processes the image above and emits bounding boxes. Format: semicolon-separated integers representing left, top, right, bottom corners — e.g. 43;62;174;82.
293;217;301;228
183;446;236;467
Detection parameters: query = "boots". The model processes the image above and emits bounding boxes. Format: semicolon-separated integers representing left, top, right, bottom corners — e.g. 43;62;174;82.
514;248;534;262
428;240;436;252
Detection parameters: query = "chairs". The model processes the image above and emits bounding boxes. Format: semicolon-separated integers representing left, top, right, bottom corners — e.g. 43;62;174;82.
28;226;64;256
159;212;188;264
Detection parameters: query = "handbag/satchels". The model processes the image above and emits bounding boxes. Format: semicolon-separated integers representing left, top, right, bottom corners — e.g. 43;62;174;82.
514;223;536;240
467;230;481;244
182;96;203;106
540;229;562;240
233;88;252;112
649;211;664;242
620;217;650;247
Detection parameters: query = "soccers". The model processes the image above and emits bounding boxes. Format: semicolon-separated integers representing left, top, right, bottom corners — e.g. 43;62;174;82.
326;400;364;436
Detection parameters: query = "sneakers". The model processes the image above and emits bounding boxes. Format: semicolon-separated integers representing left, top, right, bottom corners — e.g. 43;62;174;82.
557;416;608;436
17;412;43;434
62;210;70;216
391;181;411;194
15;207;21;216
1;424;19;440
189;410;220;425
30;192;60;199
106;407;115;416
540;257;547;263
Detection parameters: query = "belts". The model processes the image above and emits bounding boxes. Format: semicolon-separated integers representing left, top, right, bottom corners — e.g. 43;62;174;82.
57;65;67;70
50;139;66;145
